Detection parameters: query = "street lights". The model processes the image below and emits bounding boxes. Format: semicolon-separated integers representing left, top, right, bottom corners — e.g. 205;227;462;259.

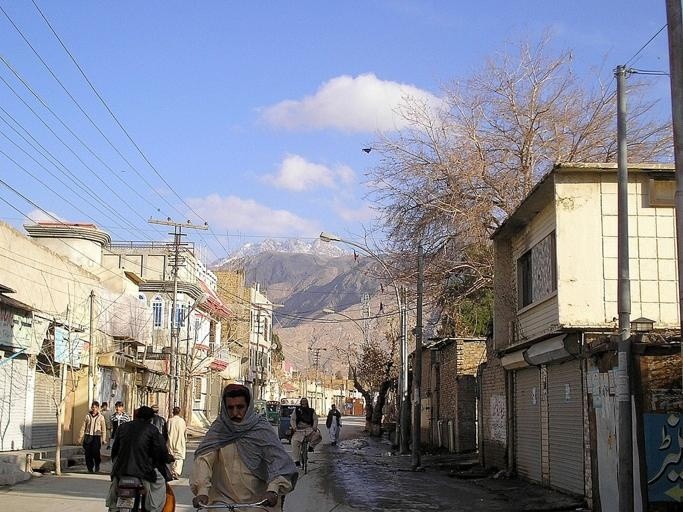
168;292;208;417
317;229;411;457
255;342;278;400
321;305;375;408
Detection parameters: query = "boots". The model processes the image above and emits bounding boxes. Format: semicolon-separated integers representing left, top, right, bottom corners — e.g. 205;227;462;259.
88;465;100;473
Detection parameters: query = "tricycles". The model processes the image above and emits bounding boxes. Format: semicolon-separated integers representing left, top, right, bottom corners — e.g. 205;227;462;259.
275;402;302;445
263;399;281;427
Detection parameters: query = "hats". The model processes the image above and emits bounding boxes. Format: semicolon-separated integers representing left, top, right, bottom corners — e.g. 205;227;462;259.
151;404;159;413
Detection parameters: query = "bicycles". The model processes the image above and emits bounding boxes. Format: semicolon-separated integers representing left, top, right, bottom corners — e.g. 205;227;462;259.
193;493;274;511
288;423;317;476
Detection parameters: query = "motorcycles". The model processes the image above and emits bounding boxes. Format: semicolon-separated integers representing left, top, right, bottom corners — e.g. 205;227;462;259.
109;453;178;512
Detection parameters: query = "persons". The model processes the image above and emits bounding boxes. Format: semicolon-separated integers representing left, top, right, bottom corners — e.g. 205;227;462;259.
165;407;188;481
98;401;113;449
325;403;343;447
107;401;130;466
77;401;106;471
151;405;167;446
290;396;322;466
105;405;182;512
188;382;300;512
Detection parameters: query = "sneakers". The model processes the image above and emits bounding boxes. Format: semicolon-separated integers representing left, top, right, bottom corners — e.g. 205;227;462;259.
171;474;179;480
295;461;301;466
308;445;315;452
